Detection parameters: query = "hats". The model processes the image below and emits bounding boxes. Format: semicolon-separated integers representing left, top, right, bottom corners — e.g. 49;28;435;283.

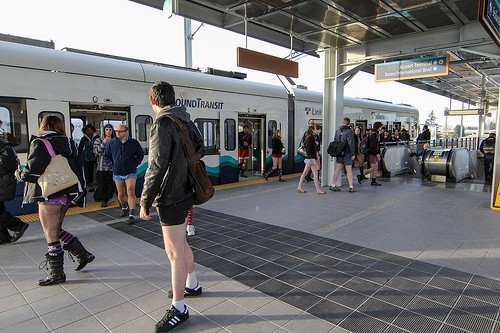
105;124;112;128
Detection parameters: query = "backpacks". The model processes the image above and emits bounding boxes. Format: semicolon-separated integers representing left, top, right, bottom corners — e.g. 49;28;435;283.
359;132;380;154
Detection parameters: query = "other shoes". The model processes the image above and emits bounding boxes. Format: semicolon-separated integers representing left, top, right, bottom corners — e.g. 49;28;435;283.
298;188;306;193
357;175;363;184
100;201;108;207
317;189;326;193
349;188;357;192
371;182;382;186
329;185;341;191
88;188;96;192
240;170;249;178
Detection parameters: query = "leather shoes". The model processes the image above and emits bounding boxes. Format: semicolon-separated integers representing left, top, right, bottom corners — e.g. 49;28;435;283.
10;223;29;242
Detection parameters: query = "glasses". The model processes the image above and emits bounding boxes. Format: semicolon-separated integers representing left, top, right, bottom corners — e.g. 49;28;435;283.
114;130;127;132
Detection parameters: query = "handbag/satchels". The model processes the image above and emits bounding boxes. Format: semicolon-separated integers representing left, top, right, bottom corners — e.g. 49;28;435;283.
297;145;307;157
327;141;346;157
33;138;79;196
316;155;321;170
188;158;214;205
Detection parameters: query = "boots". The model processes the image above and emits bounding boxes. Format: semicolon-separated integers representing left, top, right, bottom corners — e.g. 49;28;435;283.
263;168;274;180
278;168;287;182
63;236;95;272
37;251;66;287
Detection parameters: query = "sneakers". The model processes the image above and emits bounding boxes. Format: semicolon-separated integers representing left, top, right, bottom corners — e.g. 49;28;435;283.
129;215;135;224
187;226;196;236
120;208;129;216
168;285;203;298
155;304;190;333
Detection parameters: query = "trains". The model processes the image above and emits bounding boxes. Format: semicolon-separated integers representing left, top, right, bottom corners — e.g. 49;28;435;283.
0;32;421;218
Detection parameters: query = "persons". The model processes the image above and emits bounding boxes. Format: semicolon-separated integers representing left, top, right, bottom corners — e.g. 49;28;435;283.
0;120;30;245
300;124;322;183
138;79;208;333
78;124;101;193
13;115;96;287
103;124;145;224
186;206;197;236
237;125;252;178
361;124;431;145
357;121;383;187
480;133;496;182
92;123;118;208
344;126;369;182
69;121;74;139
296;126;326;194
330;117;358;194
263;129;288;182
